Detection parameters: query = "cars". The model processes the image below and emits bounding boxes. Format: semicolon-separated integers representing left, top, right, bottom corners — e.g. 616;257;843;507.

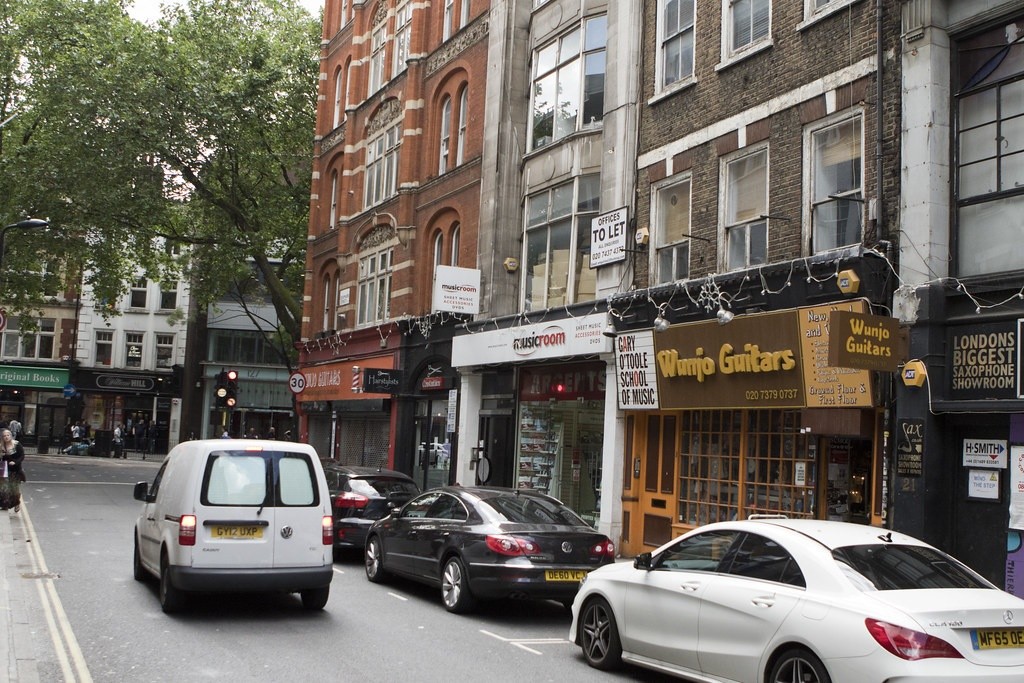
569;514;1024;683
364;486;616;614
421;442;447;464
325;465;422;557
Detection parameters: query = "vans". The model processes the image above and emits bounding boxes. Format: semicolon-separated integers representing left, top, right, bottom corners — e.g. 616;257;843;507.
133;438;334;612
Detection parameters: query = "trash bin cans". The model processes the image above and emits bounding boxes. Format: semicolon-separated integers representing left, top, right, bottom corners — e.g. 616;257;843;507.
95;429;113;457
38;436;49;454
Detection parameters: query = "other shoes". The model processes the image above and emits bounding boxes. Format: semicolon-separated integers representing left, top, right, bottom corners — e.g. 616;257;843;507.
15;505;20;512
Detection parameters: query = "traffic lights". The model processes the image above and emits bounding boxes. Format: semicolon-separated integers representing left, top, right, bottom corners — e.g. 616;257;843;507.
226;371;237;407
217;374;227;406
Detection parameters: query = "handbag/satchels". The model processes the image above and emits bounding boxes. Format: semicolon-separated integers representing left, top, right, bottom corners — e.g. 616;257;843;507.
0;459;9;478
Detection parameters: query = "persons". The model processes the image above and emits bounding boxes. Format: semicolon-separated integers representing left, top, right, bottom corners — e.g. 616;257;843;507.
0;430;25;513
221;426;291;441
10;417;157;454
